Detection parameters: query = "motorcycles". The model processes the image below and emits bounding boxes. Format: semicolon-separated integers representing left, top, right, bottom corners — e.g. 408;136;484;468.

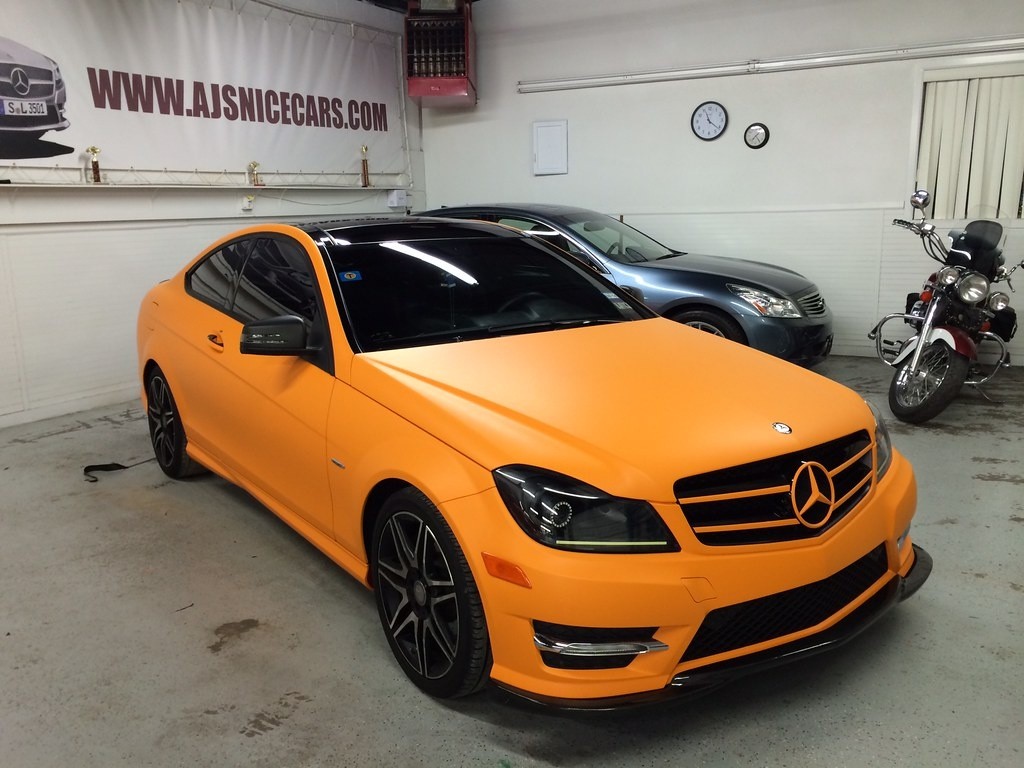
867;190;1024;424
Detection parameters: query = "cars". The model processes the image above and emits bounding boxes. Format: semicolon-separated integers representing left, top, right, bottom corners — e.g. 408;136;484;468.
1;35;70;145
411;204;834;366
135;217;935;716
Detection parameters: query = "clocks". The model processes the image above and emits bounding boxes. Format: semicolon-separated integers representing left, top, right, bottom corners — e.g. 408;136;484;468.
690;101;729;141
744;122;770;149
